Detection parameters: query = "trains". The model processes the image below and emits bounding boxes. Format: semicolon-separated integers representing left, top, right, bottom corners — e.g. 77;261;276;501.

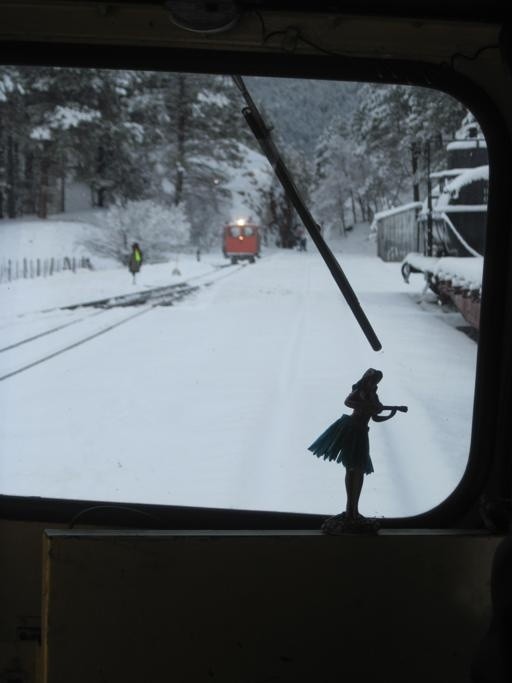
223;222;261;264
377;110;490;334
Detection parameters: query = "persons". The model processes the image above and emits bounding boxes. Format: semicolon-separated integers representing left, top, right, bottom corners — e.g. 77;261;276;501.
345;368;398;513
297;225;308;253
